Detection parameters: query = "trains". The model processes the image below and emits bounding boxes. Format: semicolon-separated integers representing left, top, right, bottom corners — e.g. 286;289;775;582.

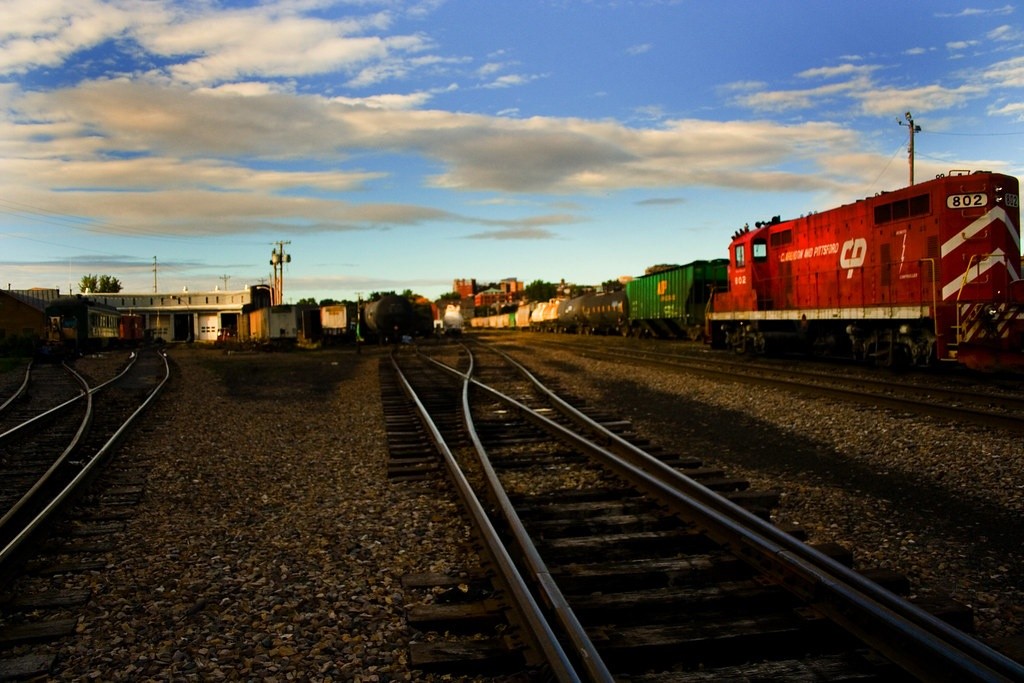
471;169;1021;382
38;298;146;353
